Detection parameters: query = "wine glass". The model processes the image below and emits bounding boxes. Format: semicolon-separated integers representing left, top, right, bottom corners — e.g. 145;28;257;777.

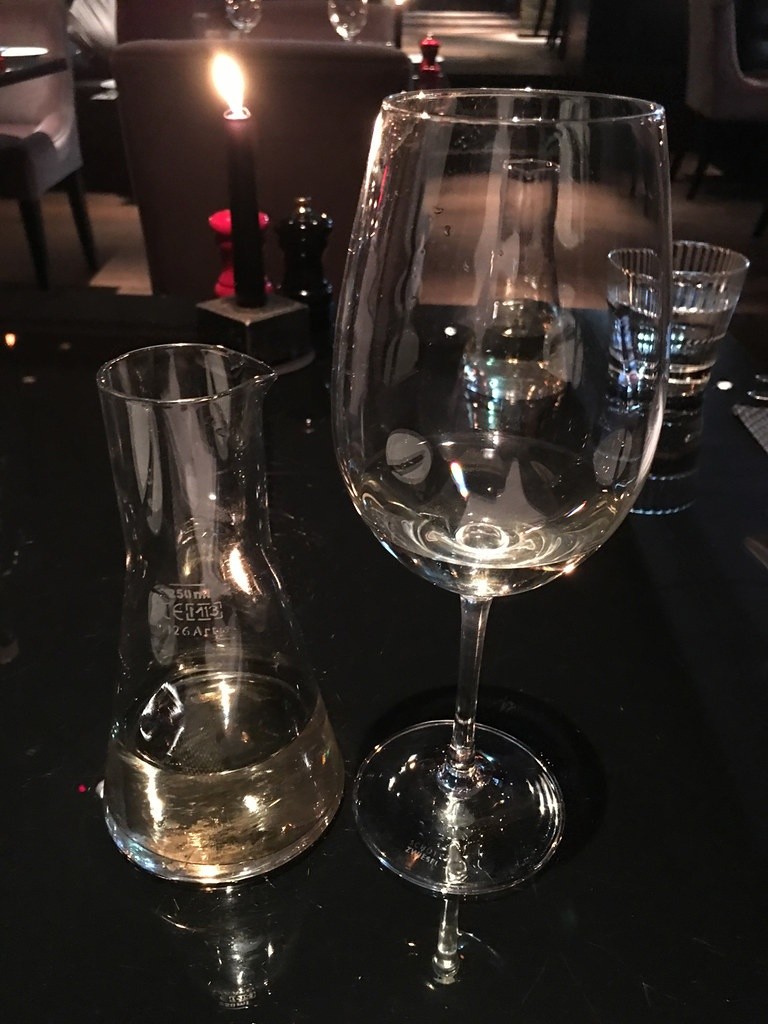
326;90;668;898
327;0;368;44
226;0;262;40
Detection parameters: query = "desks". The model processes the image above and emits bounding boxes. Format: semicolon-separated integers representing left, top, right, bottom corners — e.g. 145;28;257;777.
0;296;768;1024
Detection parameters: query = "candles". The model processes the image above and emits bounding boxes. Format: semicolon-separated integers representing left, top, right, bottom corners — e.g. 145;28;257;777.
212;52;266;308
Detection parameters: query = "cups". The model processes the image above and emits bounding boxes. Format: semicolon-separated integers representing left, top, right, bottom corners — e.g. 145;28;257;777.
96;343;344;885
610;240;749;374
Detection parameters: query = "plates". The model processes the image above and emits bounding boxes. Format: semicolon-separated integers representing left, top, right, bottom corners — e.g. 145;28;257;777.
1;47;48;56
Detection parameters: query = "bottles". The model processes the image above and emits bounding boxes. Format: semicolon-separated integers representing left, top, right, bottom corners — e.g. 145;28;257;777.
419;36;440;89
209;210;271;297
274;196;335;327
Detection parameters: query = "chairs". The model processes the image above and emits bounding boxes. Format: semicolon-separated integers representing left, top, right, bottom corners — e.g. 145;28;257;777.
111;0;415;296
0;58;97;290
669;0;768;200
